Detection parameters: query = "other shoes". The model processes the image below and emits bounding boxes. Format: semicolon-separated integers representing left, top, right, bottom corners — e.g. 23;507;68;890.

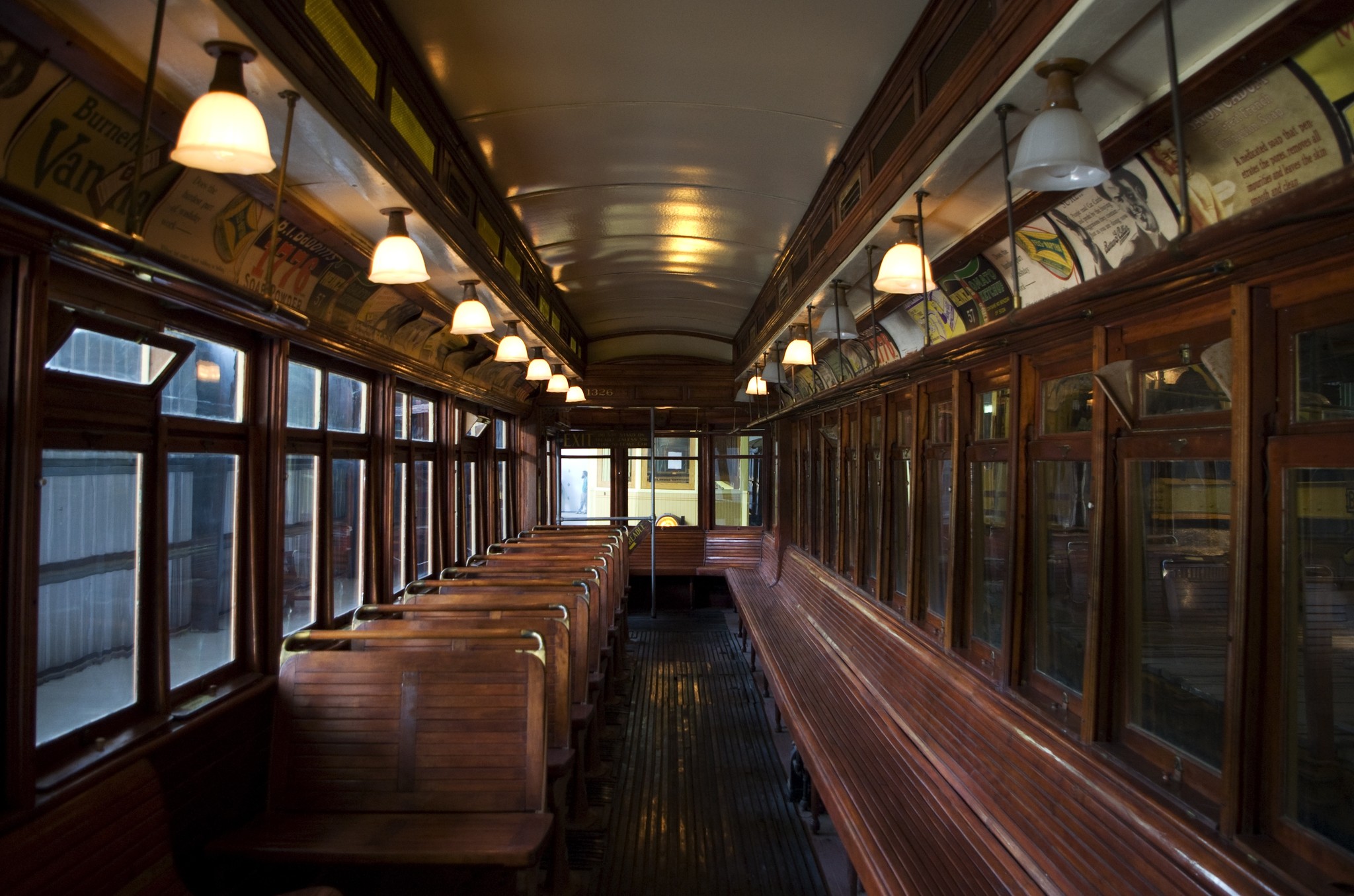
574;509;588;514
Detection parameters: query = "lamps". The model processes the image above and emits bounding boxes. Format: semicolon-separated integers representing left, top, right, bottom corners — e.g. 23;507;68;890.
872;215;939;294
450;280;495;335
494;319;530;363
781;323;817;366
369;207;432;285
565;376;587;402
546;363;570;393
745;365;769;395
526;346;553;380
815;284;859;339
169;41;277;175
734;379;756;404
1008;60;1111;191
760;347;788;383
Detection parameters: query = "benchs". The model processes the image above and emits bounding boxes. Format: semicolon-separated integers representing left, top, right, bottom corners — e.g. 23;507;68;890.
204;625;555;896
733;543;1296;896
629;520;786;638
350;602;579;896
403;525;629;834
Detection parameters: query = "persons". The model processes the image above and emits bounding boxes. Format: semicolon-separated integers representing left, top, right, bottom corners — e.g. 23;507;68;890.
575;470;588;514
1095;166;1173;268
1152;137;1225;233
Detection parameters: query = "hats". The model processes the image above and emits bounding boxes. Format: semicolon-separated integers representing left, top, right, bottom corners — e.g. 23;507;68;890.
1093;165;1147;202
